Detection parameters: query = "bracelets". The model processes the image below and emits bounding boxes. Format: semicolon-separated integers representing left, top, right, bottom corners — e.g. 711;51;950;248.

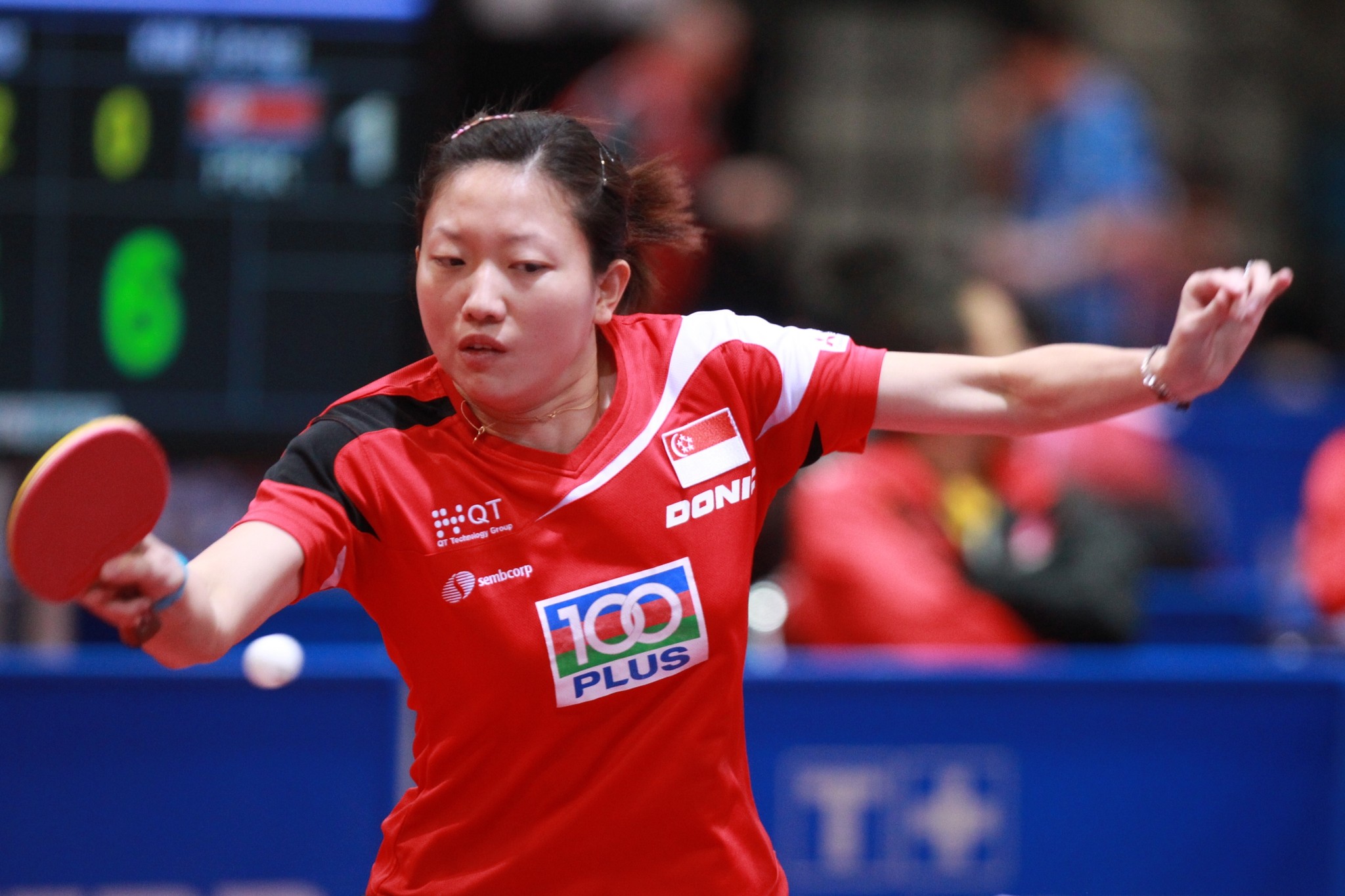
1142;345;1193;412
153;550;191;614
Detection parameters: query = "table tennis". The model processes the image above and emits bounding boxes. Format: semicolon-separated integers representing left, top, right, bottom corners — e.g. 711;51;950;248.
245;632;304;690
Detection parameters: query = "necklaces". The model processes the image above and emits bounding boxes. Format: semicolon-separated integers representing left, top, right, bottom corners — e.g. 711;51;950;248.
459;385;600;441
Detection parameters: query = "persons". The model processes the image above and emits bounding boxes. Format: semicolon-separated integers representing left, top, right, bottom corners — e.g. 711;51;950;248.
88;114;1291;895
549;1;1345;674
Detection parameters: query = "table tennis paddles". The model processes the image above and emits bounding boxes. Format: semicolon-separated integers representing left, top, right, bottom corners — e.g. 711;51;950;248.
4;414;172;603
